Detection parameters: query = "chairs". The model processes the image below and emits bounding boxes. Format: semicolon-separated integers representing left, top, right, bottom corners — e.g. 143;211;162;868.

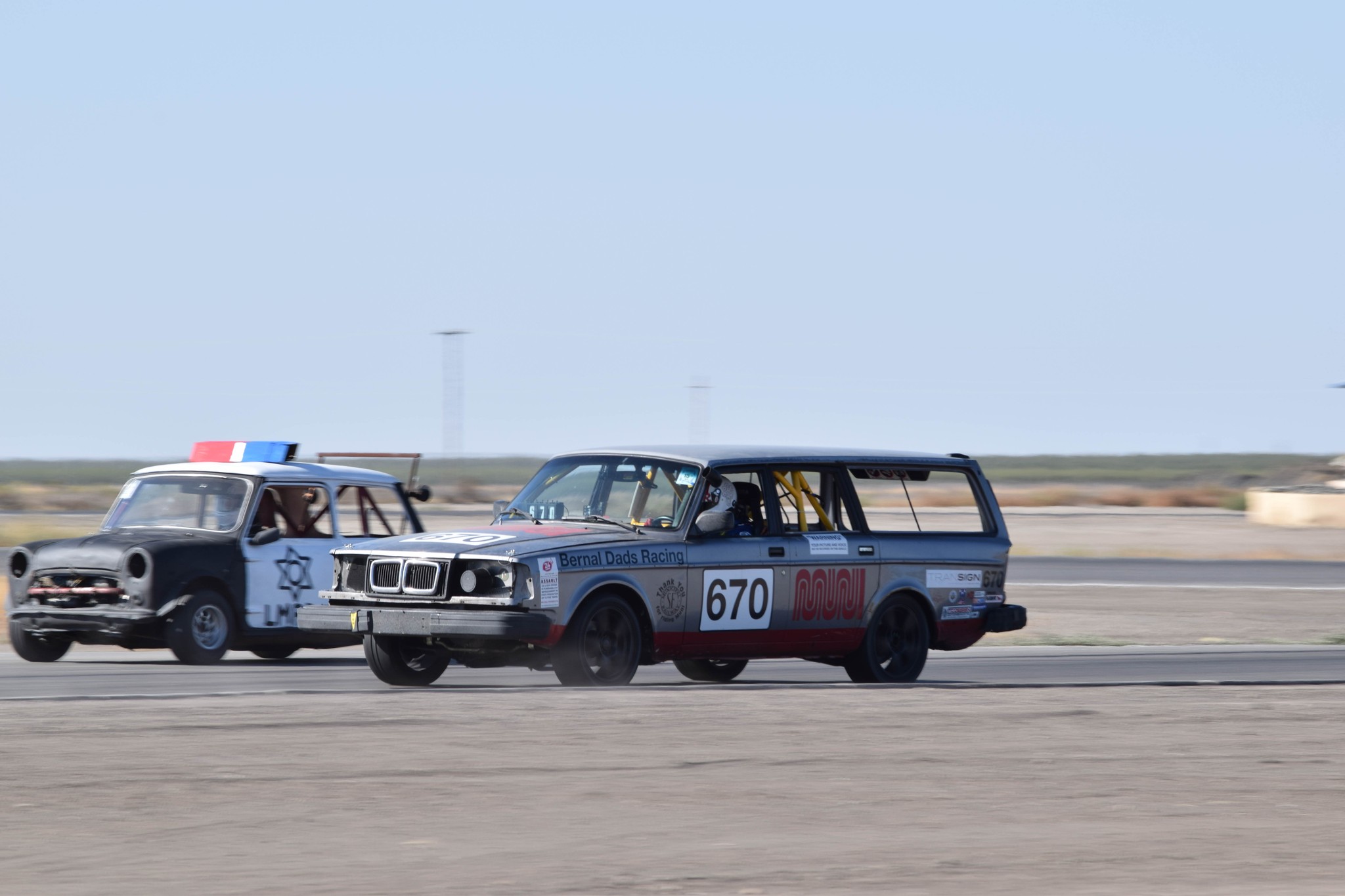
732;481;765;535
256;489;276;528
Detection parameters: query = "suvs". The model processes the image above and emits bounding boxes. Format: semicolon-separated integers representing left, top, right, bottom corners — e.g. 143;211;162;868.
319;448;1030;687
3;441;432;665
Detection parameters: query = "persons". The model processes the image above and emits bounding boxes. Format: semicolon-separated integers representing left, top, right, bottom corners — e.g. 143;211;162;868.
212;483;269;540
644;474;752;536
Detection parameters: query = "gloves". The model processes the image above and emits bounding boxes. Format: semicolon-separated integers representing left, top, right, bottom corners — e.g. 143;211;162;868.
644;518;653;526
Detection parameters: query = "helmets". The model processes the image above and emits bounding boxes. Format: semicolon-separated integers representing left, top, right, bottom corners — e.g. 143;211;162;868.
697;475;738;516
